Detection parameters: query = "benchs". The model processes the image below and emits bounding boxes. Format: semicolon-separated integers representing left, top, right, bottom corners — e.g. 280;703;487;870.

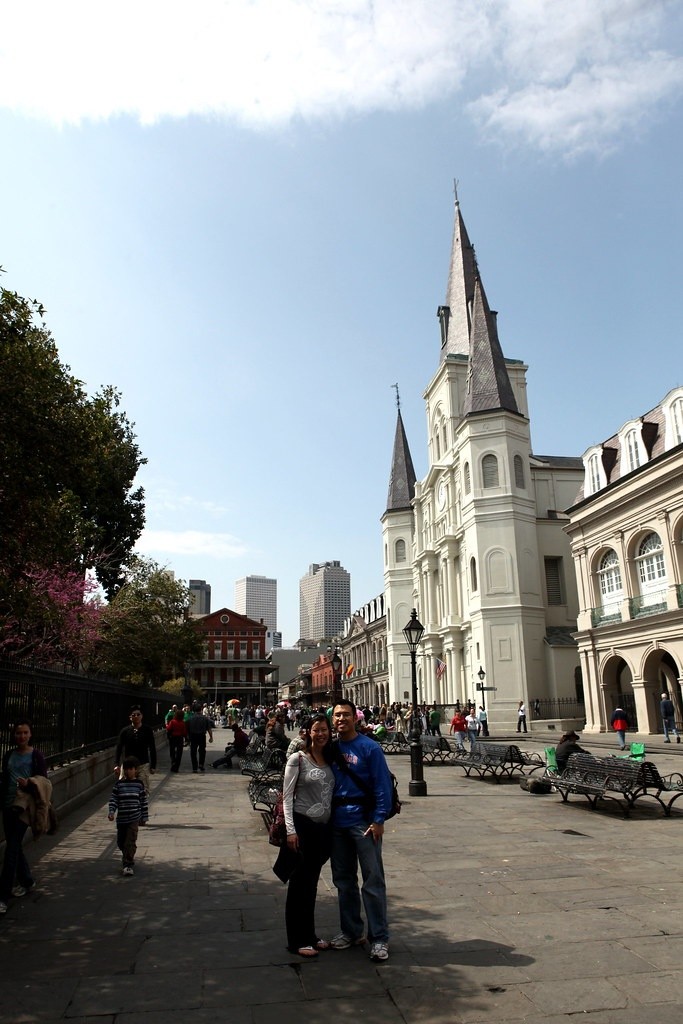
228;731;287;832
375;731;683;820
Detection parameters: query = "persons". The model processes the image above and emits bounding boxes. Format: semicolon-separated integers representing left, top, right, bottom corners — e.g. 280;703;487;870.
328;701;394;963
610;705;628;752
166;702;490;773
661;693;681;743
556;731;592;775
516;701;528;733
0;719;47;914
113;704;157;826
108;756;149;876
286;714;340;960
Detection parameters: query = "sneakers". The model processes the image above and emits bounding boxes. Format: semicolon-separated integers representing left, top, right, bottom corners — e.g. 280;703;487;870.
330;932;366;949
12;881;36;896
369;942;390;961
0;901;7;912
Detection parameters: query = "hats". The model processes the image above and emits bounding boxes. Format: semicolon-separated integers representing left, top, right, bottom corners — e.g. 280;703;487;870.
232;723;240;731
267;711;276;720
563;727;580;741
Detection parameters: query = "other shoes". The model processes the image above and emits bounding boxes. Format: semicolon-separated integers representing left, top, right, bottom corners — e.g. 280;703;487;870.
664;739;670;743
676;737;680;744
621;746;626;751
165;762;235;773
523;730;527;733
123;867;134;876
516;730;521;732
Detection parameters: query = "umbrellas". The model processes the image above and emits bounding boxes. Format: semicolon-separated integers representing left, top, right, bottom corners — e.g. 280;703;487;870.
226;699;240;706
277;701;292;708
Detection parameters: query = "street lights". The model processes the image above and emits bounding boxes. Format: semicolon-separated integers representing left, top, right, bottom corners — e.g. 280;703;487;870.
402;608;428;797
477;665;486;708
329;647;343;703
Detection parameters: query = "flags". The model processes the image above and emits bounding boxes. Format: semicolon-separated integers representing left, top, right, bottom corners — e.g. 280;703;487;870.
344;662;355;679
436;659;446;679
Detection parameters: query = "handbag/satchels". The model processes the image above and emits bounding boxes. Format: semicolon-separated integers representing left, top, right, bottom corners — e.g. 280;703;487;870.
364;773;402;826
269;756;301;847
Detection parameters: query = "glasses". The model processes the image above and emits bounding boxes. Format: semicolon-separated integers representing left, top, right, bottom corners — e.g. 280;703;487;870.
131;713;141;717
333;712;354;720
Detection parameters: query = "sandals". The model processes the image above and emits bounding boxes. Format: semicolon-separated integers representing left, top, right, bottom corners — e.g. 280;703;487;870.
314;938;331;951
288;945;318;957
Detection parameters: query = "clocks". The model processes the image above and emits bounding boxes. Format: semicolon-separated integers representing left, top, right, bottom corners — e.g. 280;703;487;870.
436;478;448;513
